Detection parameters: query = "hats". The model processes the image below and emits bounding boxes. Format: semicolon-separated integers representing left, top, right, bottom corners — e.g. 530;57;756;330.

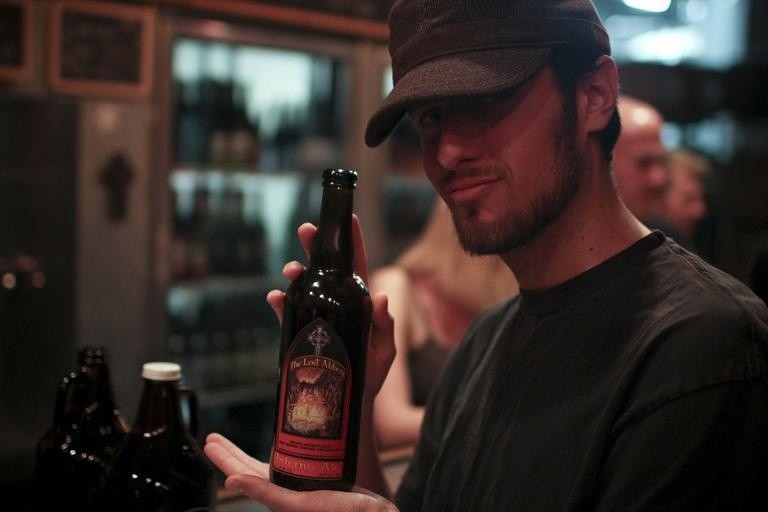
364;0;610;149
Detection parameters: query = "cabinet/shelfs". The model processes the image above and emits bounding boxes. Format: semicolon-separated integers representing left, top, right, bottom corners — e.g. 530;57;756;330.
148;18;374;404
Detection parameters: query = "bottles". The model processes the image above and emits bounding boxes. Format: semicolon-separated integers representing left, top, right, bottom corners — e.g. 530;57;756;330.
31;344;131;510
166;71;279;390
94;358;220;510
264;165;374;493
